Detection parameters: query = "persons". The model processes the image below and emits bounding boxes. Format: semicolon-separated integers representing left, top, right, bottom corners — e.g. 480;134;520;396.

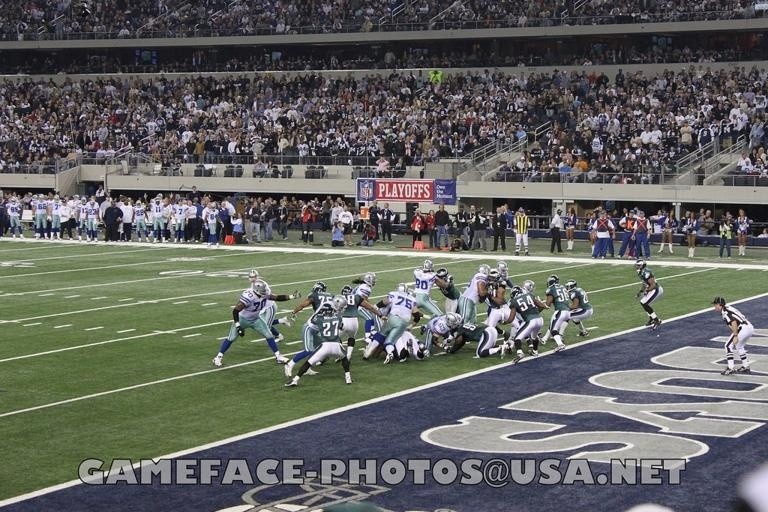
0;0;767;387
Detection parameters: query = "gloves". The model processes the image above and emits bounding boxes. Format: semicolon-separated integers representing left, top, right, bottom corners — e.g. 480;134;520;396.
236;326;244;336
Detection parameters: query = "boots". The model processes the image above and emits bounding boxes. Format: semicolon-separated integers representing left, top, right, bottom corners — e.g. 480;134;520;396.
208;243;211;248
36;232;98;242
13;235;15;239
591;245;595;254
216;243;219;248
738;245;746;256
658;243;664;253
20;234;24;239
688;246;695;258
139;238;199;244
567;241;574;249
669;242;673;254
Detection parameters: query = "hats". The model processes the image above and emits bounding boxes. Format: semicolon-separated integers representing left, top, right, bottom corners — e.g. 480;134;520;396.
711;296;725;306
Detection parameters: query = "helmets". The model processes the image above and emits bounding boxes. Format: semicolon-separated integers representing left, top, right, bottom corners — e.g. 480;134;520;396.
565;279;577;292
478;261;535;298
547;275;559;287
312;282;327;293
252;279;270;299
436;268;448;280
634;259;647;275
332;295;348;314
407;290;416;298
249;270;258;281
341;285;354;296
423;260;434;273
363;272;377;287
445;312;459;339
396;282;407;293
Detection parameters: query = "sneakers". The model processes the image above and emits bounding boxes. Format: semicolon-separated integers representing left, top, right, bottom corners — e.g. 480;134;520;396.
335;376;352;384
500;331;565;364
577;331;589;337
276;355;329;387
407;338;413;357
645;319;662;331
274;335;284;343
721;366;750;375
617;255;651;260
212;356;224;367
384;353;394;365
525;253;530;256
419;343;430;359
283;316;291;328
515;253;519;255
